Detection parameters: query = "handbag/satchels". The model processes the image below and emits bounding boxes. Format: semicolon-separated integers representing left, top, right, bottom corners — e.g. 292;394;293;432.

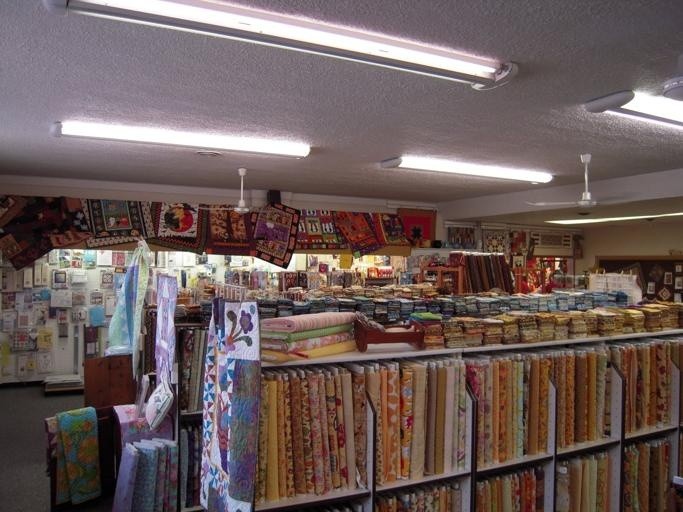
589;268;642;304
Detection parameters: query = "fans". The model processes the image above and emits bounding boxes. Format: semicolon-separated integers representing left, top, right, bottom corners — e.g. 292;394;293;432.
194;167;274;215
525;153;633;209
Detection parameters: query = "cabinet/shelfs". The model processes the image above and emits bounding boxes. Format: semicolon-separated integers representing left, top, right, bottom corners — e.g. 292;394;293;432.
254;325;682;510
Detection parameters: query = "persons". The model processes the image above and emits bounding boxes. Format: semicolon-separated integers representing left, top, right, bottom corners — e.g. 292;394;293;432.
530;270;565;294
381;215;403;242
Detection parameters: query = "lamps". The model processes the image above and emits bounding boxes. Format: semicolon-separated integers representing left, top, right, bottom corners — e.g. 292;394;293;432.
380;155;553;186
52;0;516;91
51;119;310;160
583;89;682;133
